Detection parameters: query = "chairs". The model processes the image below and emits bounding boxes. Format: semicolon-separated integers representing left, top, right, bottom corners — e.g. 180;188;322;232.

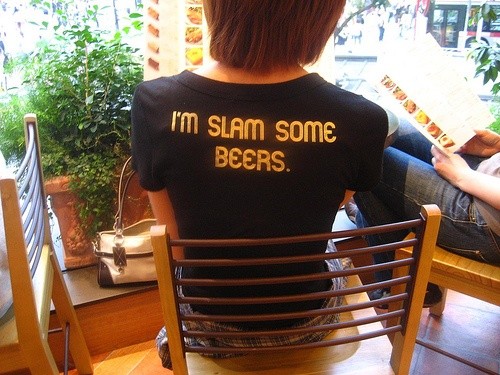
386;232;500;375
150;202;441;375
0;112;96;375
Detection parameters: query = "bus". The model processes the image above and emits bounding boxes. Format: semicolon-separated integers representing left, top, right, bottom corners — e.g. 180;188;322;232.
410;0;500;56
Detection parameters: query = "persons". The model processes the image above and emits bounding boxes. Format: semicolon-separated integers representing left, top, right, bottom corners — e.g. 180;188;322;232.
130;0;390;350
344;116;500;309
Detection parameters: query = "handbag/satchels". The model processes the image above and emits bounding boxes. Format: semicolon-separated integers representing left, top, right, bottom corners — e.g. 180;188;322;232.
91;156;161;287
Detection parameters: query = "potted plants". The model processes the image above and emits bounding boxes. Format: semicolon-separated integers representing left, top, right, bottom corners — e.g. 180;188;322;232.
0;0;156;271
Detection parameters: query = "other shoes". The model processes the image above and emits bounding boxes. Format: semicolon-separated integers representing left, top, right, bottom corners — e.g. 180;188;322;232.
369;281;443;310
345;202;365;240
